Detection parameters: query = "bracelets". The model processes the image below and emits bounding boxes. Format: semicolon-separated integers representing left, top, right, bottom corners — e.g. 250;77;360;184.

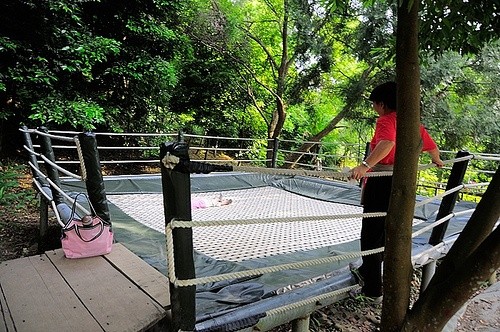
362;160;372;170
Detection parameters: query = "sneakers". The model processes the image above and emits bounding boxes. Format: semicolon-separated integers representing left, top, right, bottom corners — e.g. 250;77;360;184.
348;288;383;305
349;260;366;286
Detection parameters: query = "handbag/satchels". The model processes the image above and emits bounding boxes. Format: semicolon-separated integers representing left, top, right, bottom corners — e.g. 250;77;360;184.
60;193;113;259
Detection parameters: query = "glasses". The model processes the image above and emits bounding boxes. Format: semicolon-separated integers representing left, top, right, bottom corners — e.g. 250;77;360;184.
368;102;381;108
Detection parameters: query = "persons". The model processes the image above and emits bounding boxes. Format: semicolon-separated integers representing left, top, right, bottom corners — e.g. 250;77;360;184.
347;81;445;305
